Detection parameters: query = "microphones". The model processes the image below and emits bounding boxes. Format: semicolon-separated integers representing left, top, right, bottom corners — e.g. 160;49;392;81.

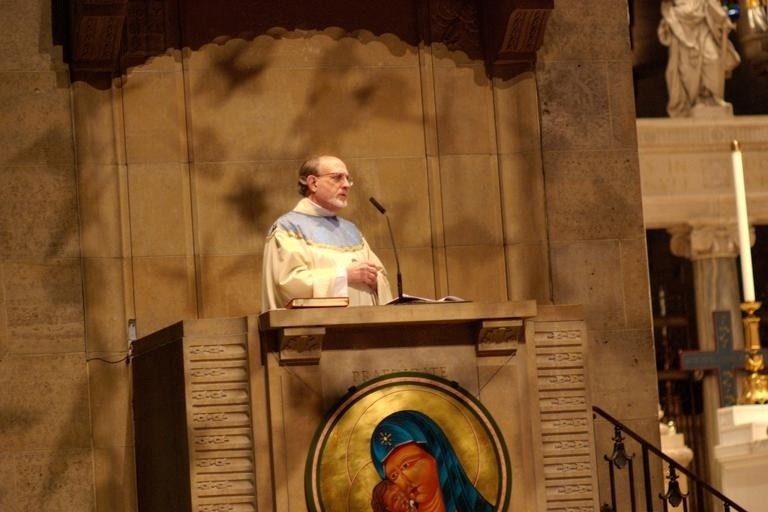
369;196;417;304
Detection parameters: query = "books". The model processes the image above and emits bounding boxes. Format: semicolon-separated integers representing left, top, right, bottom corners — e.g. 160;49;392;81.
384;295;467;303
285;296;350;310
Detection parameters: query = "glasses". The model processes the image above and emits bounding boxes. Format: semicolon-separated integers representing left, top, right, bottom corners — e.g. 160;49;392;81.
318;172;355;186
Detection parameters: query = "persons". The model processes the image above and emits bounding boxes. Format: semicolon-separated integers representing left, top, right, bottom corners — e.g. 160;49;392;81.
371;480;414;512
261;155;397;310
370;409;497;512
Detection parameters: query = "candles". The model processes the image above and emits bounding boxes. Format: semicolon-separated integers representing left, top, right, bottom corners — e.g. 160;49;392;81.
660;288;668;334
729;140;755;302
